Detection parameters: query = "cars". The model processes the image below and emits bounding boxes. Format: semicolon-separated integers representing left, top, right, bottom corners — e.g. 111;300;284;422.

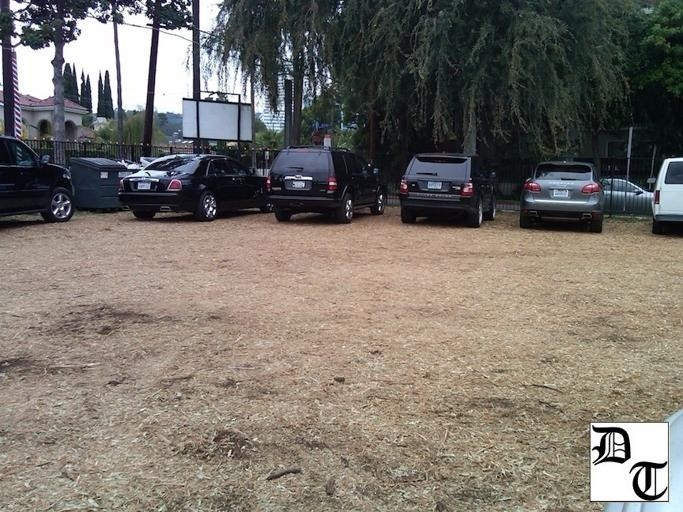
118;154;275;221
519;160;605;232
0;135;76;223
647;157;683;233
600;178;653;214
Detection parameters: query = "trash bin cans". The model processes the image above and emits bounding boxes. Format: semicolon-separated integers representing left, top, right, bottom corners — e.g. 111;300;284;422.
69;157;158;214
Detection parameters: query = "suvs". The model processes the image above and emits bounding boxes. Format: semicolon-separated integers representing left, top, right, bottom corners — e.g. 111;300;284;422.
266;145;388;224
399;153;496;228
